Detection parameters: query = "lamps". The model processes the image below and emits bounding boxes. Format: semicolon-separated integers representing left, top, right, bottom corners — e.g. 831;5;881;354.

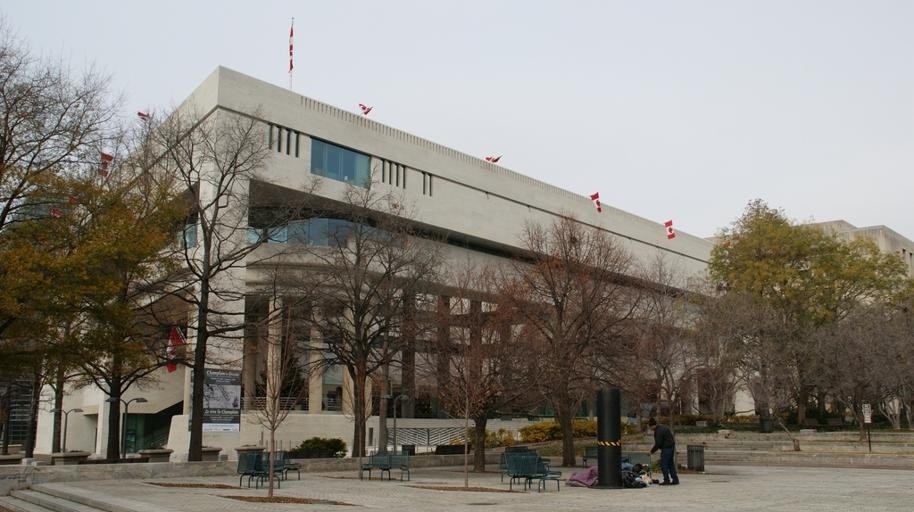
358;450;410;481
237;449;303;489
499;452;562;493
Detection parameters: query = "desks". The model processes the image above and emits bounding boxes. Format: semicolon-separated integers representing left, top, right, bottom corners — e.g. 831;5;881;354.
105;397;147;462
48;407;84;450
392;394;408;455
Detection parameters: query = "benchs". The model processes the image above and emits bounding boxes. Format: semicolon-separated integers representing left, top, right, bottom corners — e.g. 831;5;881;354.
589;191;603;214
287;25;294;72
358;103;372;116
484;154;501;164
67;195;77;206
162;324;185;376
664;219;676;239
137;111;153;125
96;152;113;177
50;206;63;219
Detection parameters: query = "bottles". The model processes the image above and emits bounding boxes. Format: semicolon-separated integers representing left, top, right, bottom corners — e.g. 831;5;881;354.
649;418;656;426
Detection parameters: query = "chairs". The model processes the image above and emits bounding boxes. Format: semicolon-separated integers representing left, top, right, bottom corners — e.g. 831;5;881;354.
671;479;679;484
659;480;670;485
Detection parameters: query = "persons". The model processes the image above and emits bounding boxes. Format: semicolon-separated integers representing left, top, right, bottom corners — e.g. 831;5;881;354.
644;416;680;484
621;461;642;476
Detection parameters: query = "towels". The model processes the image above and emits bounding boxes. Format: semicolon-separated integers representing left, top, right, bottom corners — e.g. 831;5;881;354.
402;444;415;456
687;444;704;472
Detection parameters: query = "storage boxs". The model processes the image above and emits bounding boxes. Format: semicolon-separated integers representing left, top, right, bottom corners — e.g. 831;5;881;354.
435;444;471;454
806;417;852;432
